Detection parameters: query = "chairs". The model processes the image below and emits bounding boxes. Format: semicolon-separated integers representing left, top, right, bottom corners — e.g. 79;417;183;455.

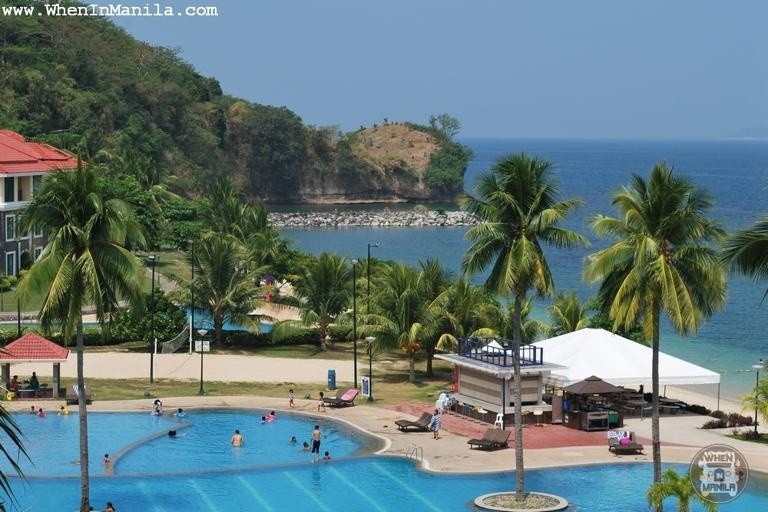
322;387;348;406
479;430;512;451
606;431;628;455
331;388;360;409
466;428;496;450
622;432;644;453
395;391;544;434
8;378;93;405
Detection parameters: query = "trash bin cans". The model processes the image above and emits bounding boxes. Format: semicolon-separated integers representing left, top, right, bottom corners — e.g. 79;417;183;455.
328;370;335;390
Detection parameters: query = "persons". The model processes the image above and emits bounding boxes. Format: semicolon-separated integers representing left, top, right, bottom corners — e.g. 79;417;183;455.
37;408;46;421
230;429;244;447
259;416;269;424
30;406;37;416
176;408;186;418
310;425;322;464
289;389;295;408
105;502;115;512
322;451;332;460
103;454;112;465
59;406;69;415
150;399;165;416
303;442;311;452
264;410;276;424
428;409;441;440
168;430;177;438
317;391;326;412
9;371;42;398
289;436;297;444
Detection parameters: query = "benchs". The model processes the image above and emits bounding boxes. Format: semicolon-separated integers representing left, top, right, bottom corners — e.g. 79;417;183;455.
545;388;687;429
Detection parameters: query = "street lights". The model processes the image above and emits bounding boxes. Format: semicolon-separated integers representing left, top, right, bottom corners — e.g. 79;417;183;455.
146;253;156;383
195;323;209;397
750;358;767;437
364;238;383;356
351;256;360;389
365;335;378;403
186;238;197;354
